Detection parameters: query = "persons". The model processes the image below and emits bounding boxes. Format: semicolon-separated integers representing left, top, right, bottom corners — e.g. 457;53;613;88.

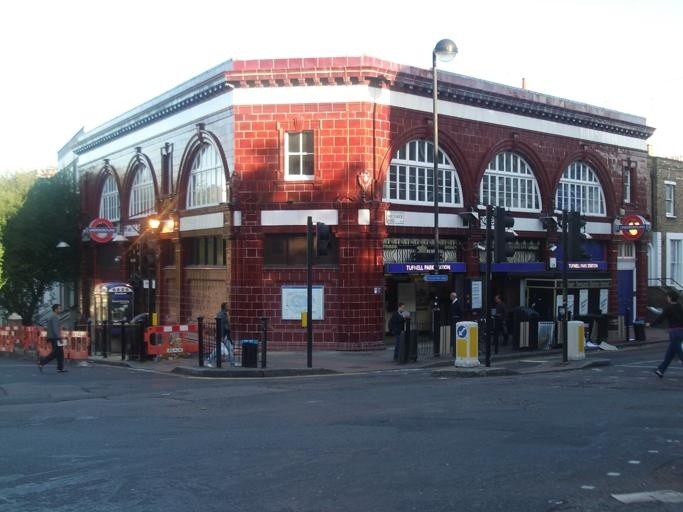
36;303;68;374
447;291;463;351
489;295;508;346
643;290;682;377
201;300;241;367
387;302;409;363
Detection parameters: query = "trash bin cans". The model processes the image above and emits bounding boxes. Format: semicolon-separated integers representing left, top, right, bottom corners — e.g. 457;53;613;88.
242;340;259;368
634;319;645;340
576;314;608;344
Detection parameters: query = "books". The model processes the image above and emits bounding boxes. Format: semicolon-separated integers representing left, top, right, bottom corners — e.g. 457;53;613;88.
56;338;67;346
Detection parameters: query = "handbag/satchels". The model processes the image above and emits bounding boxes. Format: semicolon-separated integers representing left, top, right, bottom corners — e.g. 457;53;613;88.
220;342;228;356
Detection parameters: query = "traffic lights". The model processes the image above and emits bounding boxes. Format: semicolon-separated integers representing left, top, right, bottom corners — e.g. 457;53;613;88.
315;221;332;258
567;211;586;260
493;207;515;263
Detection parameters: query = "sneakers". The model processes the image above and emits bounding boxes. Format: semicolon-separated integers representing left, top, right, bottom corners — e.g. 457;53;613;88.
203;361;213;368
231;363;241;367
656;369;663;377
37;360;68;373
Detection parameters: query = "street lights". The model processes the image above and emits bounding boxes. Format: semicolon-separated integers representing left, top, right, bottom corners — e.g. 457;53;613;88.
432;38;457;357
148;210;183;322
